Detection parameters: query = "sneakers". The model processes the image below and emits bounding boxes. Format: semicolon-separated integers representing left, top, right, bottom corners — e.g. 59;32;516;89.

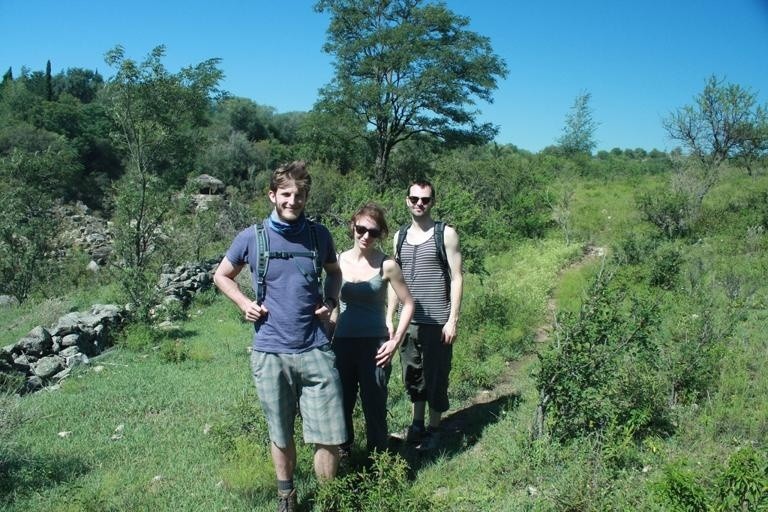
337;446;351;458
421;431;443;451
277;487;298;512
407;424;425;449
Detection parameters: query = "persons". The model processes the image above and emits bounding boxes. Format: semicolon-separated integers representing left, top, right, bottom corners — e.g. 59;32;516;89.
210;158;349;511
390;178;462;454
323;201;416;468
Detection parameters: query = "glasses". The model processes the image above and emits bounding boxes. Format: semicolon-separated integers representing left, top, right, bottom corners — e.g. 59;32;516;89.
353;224;381;238
407;196;432;206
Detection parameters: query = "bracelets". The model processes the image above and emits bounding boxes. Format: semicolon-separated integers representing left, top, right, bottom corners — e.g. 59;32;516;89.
323;296;337;309
